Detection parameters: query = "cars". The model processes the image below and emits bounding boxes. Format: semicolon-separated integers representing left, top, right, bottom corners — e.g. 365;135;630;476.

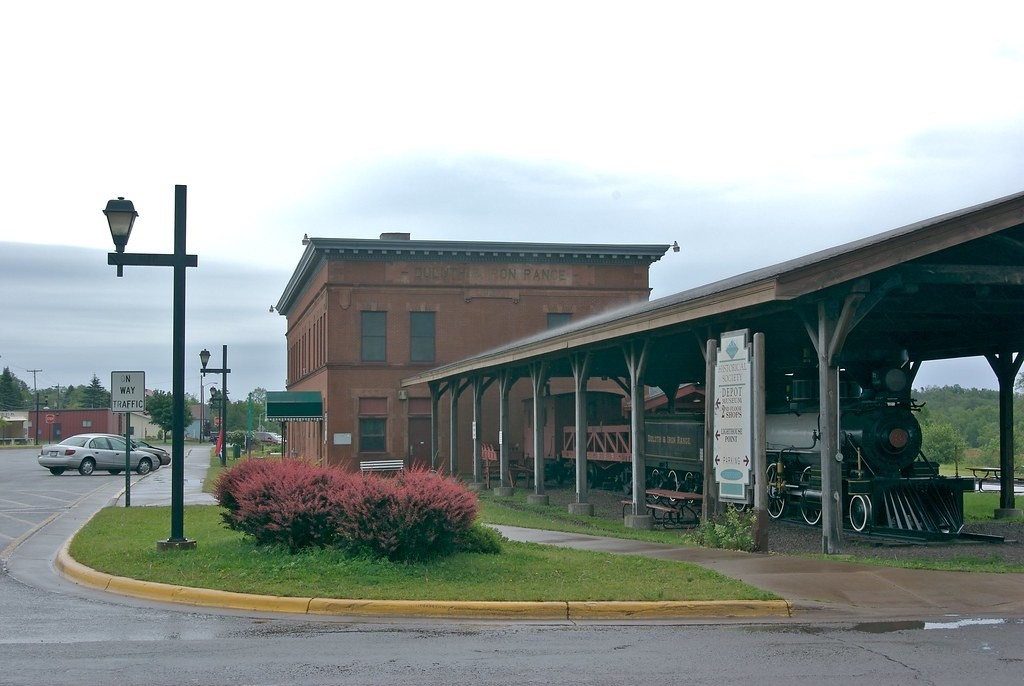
95;432;171;470
37;434;160;476
253;431;287;446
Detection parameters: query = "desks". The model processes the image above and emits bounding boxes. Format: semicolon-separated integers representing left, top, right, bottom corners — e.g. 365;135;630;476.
645;489;703;529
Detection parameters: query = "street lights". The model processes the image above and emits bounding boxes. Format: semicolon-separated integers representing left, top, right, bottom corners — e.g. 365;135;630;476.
209;385;229;434
200;344;231;467
103;183;202;550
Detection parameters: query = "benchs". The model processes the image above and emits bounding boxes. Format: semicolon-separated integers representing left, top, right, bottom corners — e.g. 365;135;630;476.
965;467;1018;493
691;504;702;514
621;500;680;529
360;460;404;477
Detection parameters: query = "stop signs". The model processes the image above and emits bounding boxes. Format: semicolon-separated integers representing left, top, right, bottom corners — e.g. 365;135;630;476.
46;414;55;423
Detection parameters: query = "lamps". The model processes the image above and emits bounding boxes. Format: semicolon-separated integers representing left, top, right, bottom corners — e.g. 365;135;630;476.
269;305;277;312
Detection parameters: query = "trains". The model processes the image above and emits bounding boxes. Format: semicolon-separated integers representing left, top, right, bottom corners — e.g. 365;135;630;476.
519;339;975;534
